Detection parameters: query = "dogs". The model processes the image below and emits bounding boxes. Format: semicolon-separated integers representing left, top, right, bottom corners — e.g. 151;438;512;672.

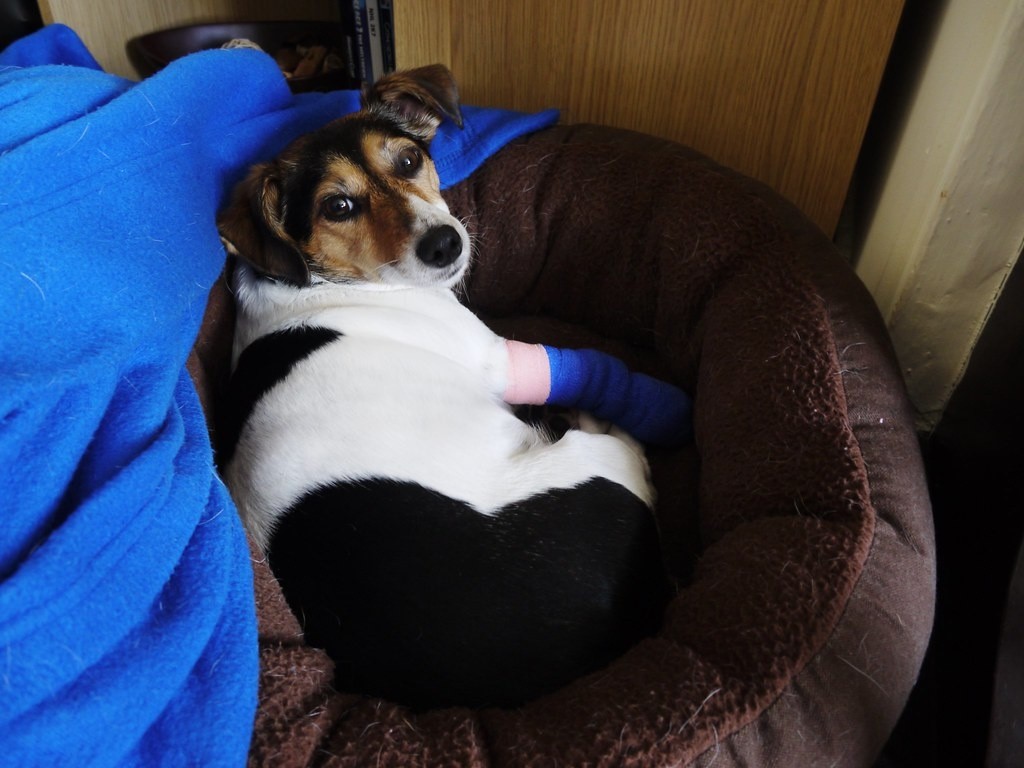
213;62;694;709
